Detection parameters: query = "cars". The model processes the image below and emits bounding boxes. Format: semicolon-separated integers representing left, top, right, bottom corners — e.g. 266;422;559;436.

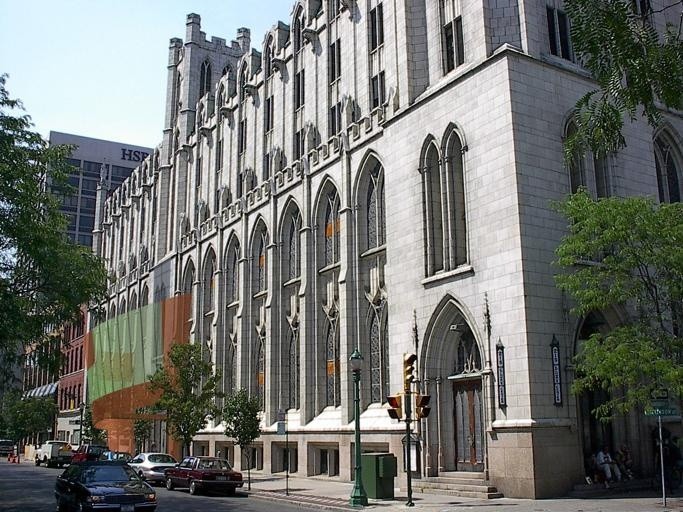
34;440;244;512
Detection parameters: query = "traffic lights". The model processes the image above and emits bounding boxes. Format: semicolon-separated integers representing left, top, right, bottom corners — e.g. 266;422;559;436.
386;395;402;419
404;353;417;384
417;395;431;417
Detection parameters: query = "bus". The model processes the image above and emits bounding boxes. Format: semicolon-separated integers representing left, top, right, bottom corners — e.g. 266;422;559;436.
0;439;14;457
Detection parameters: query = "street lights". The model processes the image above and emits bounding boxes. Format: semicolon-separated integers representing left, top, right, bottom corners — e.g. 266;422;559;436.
79;401;85;446
348;348;368;508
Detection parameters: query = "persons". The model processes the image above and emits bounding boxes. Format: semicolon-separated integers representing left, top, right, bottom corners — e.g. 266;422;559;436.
650;417;683;496
585;445;634;488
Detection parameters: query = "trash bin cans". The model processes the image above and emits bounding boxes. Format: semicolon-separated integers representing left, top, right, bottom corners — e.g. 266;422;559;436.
360;452;397;499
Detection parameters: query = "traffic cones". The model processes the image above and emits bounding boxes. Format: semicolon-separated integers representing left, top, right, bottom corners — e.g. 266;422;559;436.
7;454;20;464
